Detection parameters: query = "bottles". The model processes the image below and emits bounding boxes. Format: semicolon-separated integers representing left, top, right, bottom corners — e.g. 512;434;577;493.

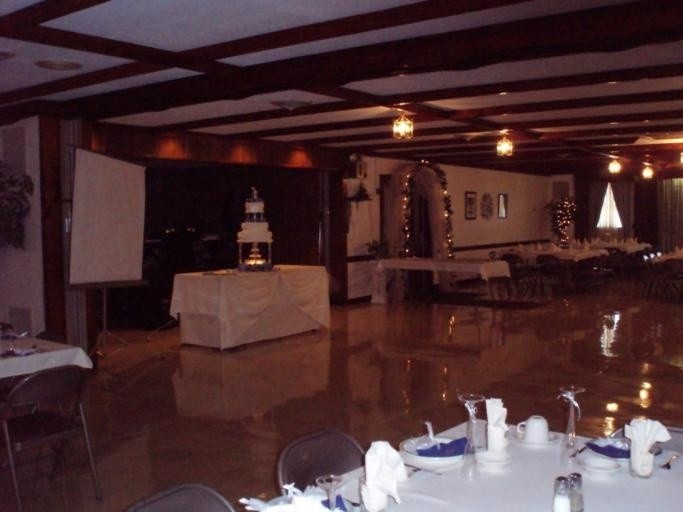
553;473;583;512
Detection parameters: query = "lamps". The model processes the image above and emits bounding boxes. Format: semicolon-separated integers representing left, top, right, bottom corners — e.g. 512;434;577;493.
494;134;514;156
393;112;413;141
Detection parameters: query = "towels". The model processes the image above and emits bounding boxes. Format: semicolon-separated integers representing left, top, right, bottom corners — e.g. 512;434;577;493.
359;435;409;512
624;416;673;478
475;395;513;478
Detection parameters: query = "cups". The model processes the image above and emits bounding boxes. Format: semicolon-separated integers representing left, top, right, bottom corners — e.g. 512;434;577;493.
359;477;389;512
457;392;512;483
632;442;656;477
518;415;550;438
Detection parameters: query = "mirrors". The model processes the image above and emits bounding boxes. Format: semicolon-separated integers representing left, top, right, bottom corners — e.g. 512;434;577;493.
498;193;508;219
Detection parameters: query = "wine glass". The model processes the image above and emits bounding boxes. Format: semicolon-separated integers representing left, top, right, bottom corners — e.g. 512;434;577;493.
1;329;29;354
316;474;348;510
561;384;586;468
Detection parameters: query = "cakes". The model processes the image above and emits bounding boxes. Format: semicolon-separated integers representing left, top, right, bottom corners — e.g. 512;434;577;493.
237;187;273;241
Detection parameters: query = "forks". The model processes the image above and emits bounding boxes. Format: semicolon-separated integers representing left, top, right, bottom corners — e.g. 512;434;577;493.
663;455;678;470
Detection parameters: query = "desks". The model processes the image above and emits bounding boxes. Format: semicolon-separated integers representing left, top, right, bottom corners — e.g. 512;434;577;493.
174;264;328;353
482;237;683;307
169;335;331;419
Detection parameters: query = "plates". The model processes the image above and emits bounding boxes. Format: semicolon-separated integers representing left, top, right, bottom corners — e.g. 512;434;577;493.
397;436;468;468
584;457;622;474
588;436;663;463
506;433;561;446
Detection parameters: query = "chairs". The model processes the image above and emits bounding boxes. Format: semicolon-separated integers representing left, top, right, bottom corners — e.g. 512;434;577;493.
31;326;99;362
276;427;368;495
0;364;102;512
123;481;235;512
606;424;682;457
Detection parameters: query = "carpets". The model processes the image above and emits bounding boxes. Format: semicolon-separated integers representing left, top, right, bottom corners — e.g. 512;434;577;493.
408;289;546;311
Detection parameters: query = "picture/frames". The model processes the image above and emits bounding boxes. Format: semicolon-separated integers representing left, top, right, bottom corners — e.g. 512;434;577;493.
464;190;477;220
